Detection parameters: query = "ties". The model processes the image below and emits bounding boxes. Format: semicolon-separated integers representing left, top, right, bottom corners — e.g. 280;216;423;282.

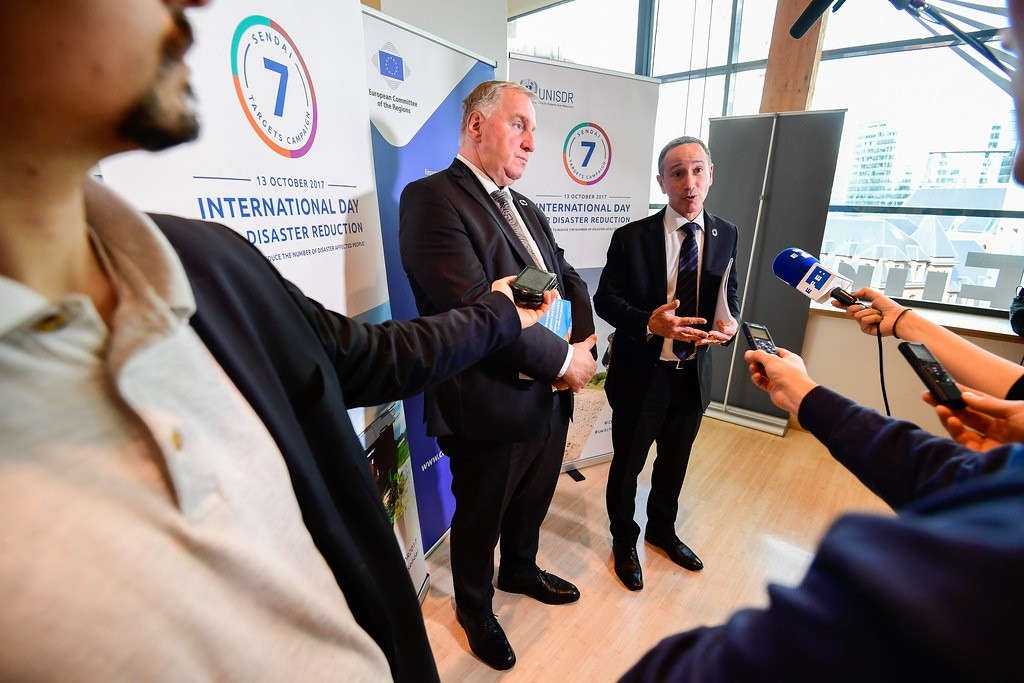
673;222;699;361
490;190;545;272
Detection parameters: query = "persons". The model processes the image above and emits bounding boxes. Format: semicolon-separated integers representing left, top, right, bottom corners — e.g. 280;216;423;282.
593;136;743;591
831;287;1024;400
920;383;1024;453
615;0;1024;683
398;81;598;670
1;0;559;683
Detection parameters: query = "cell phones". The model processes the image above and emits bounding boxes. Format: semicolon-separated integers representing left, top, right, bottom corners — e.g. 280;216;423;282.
509;266;556;297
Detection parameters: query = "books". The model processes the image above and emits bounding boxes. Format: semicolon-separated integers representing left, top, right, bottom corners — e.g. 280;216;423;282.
712;257;734;331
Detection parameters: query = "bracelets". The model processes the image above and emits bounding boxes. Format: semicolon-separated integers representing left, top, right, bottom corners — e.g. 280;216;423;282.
892;308;913;339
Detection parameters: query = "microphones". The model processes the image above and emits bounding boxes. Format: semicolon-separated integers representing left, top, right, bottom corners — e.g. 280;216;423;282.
772;247;879;325
789;0;833;40
889;0;929;11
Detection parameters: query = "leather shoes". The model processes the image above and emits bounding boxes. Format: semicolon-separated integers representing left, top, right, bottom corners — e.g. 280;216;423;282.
612;543;643;591
455;607;516;671
498;569;580;605
644;532;703;571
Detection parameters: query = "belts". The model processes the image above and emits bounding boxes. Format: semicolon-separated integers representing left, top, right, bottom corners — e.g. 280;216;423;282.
653;361;698;373
